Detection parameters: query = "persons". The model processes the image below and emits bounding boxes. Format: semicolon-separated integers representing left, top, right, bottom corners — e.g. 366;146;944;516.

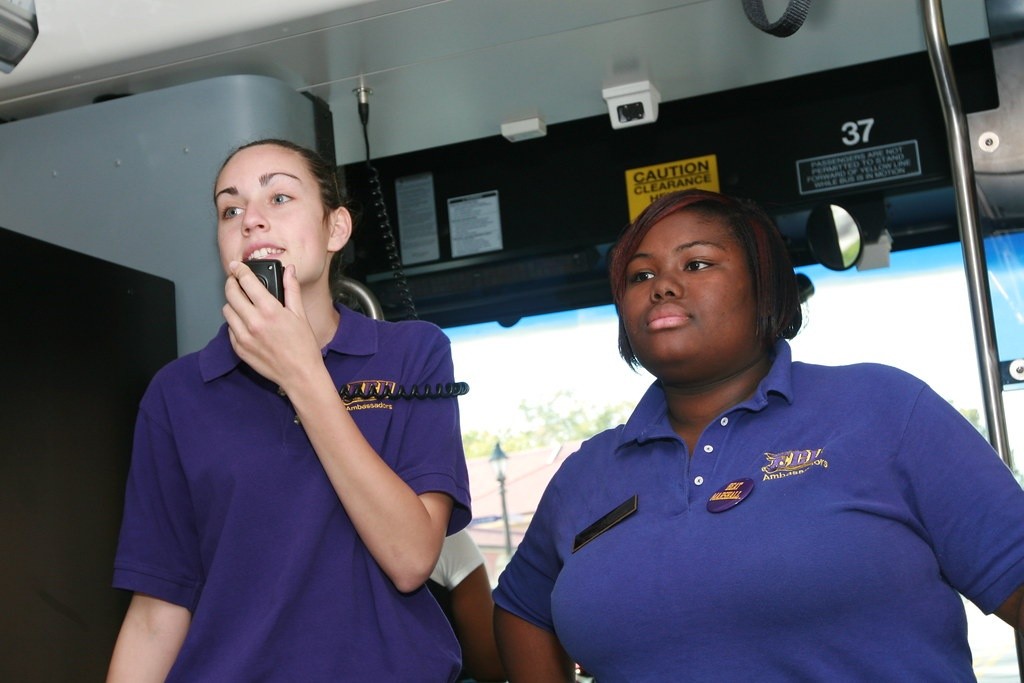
424;529;509;683
492;188;1024;683
104;137;473;683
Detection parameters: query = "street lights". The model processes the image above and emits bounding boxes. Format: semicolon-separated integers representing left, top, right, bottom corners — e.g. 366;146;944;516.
487;442;513;560
